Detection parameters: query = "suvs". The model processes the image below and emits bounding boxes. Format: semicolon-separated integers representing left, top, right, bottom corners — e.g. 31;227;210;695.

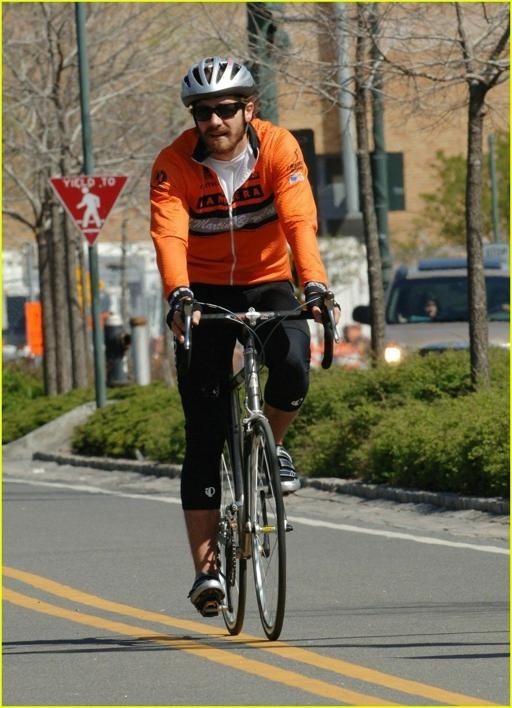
361;255;511;367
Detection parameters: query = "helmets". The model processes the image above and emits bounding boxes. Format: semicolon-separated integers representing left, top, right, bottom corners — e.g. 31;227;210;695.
181;56;255;108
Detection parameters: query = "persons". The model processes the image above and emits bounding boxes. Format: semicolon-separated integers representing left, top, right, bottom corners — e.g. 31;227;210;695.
414;292;449;322
152;55;340;618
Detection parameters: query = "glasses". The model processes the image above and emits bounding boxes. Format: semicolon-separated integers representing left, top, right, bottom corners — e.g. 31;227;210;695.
190;101;244;119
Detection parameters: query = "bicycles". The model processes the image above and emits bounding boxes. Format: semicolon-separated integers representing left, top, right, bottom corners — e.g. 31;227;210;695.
165;282;343;641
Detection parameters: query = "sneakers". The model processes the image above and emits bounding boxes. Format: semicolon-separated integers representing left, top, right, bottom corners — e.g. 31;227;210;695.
188;575;224;610
277;447;299;492
